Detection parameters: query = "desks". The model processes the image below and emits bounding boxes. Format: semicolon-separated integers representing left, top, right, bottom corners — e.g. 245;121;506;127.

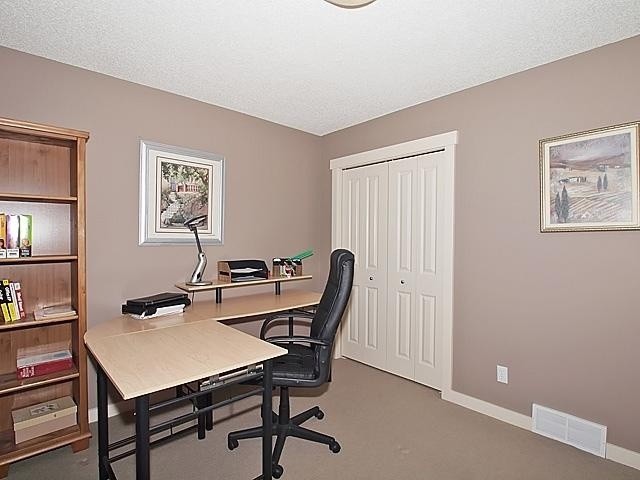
83;285;326;479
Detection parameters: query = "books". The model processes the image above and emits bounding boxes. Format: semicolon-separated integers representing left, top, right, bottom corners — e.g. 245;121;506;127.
0;213;78;380
127;292;188;305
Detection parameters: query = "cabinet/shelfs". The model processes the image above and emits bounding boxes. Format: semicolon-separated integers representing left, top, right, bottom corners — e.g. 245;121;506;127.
0;116;97;480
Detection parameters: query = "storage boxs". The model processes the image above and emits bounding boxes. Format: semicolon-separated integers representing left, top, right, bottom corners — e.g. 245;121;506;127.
9;396;81;447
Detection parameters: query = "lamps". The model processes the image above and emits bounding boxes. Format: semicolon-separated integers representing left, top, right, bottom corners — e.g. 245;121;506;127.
184;213;216;289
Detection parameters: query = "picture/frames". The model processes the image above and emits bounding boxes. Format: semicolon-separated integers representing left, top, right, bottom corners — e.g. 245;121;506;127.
535;119;640;234
138;137;225;247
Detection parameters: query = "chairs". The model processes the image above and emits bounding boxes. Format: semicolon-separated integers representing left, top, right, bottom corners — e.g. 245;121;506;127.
223;246;359;479
